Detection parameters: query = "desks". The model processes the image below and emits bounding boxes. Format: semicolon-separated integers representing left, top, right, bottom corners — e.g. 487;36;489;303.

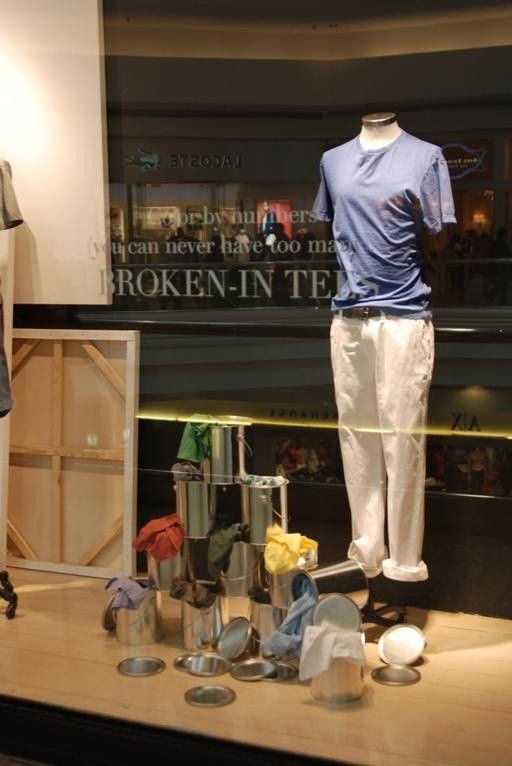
0;566;512;766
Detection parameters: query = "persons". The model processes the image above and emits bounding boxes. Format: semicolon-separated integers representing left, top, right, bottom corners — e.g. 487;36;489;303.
307;112;458;582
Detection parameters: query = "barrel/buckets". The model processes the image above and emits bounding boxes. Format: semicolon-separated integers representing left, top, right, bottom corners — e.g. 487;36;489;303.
203;427;251;485
175;479;217;541
241;474;289;548
182;600;224;652
264;548;320;610
247;597;291;661
213;542;265;594
112;590;160;644
146;546;188;589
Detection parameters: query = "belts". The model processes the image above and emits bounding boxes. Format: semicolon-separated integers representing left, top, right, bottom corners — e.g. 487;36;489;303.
338;307;383;319
